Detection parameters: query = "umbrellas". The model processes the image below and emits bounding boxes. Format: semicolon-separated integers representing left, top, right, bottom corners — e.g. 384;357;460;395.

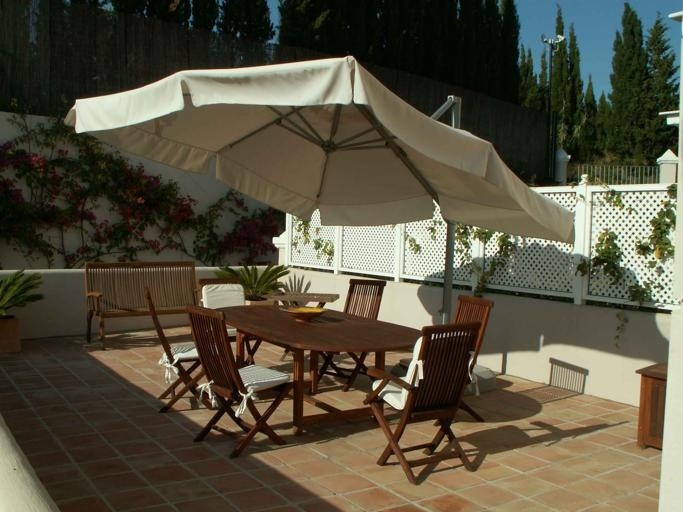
63;56;577;395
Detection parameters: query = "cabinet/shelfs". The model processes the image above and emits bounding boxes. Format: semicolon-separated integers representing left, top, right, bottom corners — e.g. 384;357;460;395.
636;360;667;452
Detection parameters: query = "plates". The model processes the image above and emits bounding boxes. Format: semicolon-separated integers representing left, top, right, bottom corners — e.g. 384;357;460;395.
280;306;327;324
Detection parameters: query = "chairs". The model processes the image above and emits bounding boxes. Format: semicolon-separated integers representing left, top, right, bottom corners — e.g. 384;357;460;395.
143;284;214;413
362;320;482;484
195;277;264;364
308;278;387;393
400;293;495;424
185;303;295;459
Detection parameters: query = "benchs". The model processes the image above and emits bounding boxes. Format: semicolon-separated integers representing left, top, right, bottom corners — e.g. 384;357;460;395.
84;260;197;352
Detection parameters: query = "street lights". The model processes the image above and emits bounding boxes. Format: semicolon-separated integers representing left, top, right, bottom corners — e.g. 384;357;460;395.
540;34;565;186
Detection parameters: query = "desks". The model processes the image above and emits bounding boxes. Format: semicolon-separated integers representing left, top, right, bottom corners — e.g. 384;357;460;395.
261;292;340;309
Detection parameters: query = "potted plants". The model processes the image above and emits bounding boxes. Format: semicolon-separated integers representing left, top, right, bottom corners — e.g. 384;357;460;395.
0;267;46;353
